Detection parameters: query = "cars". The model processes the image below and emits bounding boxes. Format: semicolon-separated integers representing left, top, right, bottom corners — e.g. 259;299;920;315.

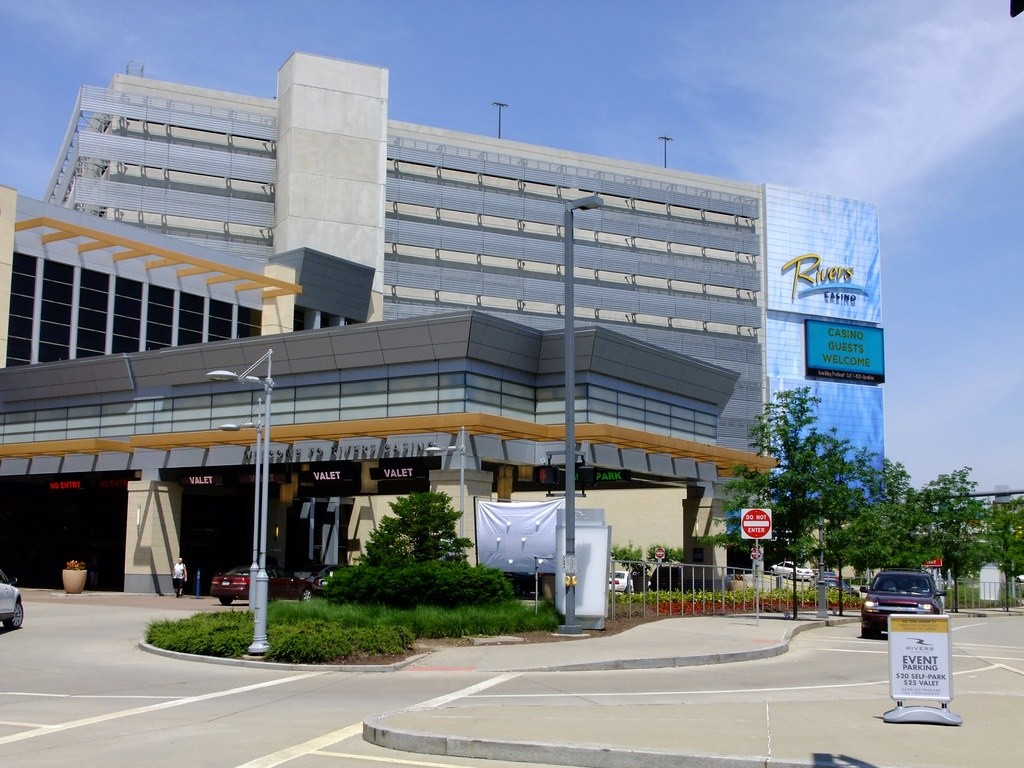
211;564;313;604
809;570;860;599
609;570;651;593
769;561;814;582
0;568;25;629
294;563;346;594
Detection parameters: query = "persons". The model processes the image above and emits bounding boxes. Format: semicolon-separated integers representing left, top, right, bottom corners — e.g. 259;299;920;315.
173;558;187;598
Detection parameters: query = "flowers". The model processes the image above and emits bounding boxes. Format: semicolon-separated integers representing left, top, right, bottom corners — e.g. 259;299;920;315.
66;560;86;571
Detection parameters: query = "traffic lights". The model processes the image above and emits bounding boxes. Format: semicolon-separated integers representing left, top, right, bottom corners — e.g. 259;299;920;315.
578;466;597;487
532;465;559;485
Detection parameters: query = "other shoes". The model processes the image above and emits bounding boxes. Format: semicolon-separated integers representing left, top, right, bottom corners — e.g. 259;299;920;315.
176;593;182;597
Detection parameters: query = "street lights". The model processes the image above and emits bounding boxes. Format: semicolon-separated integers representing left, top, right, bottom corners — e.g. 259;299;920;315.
219;398;264;611
553;195;605;635
492;102;509;139
203;347;275;655
658;135;674;168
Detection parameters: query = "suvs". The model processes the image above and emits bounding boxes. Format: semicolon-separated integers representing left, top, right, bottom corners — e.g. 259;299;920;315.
858;568;947;639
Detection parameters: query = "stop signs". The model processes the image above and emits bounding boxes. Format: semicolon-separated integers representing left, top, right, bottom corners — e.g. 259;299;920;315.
654;547;665;559
741;508;772;541
750;548;762;560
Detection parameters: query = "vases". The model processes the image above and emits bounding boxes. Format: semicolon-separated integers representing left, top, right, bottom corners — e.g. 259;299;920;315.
62;570;87;594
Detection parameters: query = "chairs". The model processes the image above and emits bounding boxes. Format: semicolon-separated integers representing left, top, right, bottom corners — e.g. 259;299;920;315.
883;580;896;591
